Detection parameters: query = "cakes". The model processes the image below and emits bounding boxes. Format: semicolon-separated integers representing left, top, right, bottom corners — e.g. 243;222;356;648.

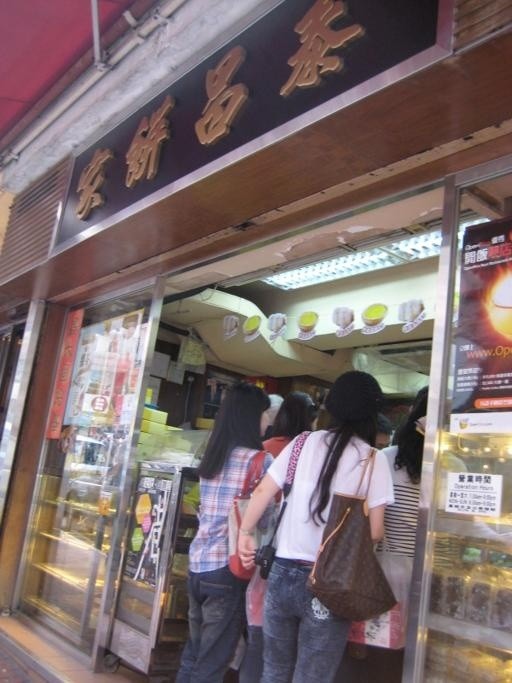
425;571;512;683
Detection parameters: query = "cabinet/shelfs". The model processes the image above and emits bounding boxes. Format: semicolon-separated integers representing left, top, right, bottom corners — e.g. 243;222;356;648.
419;423;512;683
40;457;198;675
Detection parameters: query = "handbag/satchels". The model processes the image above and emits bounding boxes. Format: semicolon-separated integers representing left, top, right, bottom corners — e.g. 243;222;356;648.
228;497;286;581
306;493;397;621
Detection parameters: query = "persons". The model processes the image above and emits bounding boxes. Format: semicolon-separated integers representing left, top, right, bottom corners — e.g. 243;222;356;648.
174;370;474;682
